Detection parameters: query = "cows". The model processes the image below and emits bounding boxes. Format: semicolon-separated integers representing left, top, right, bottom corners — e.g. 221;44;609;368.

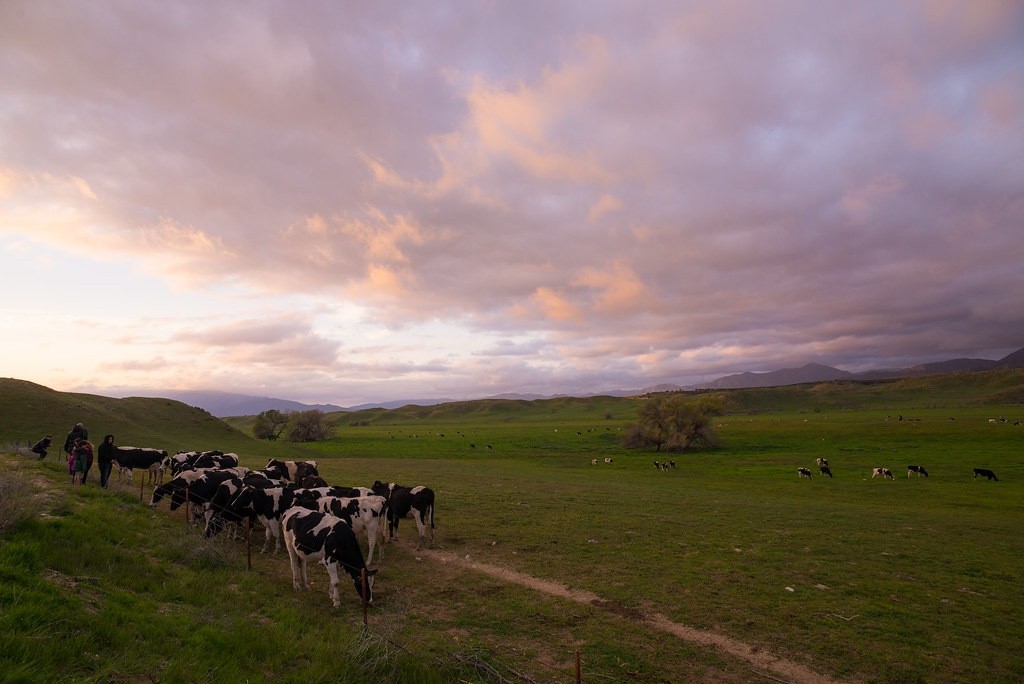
605;457;613;464
973;468;998;481
797;457;833;480
907;465;928;479
113;446;435;608
592;458;598;466
871;467;895;481
654;460;675;472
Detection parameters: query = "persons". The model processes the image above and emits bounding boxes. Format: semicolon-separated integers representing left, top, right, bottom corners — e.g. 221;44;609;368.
98;435;115;489
32;434;52;460
68;438;93;485
64;423;88;451
